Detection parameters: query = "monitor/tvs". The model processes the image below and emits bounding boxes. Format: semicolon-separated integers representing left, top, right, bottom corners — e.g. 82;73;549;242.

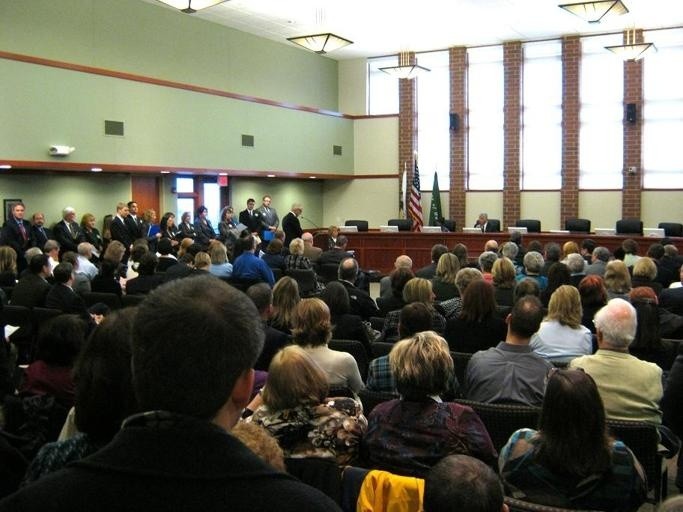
422;226;441;233
646;228;663;240
463;228;482;234
380;225;398;232
508;227;527;234
337;226;358;233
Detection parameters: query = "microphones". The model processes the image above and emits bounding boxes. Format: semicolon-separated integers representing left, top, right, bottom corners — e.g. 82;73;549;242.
301;216;321;233
474;220;479;228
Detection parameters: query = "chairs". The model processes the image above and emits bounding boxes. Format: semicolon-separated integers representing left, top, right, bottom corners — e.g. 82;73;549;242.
432;220;457;234
656;222;683;237
474;220;501;233
1;267;683;512
516;220;541;233
616;220;644;235
388;220;413;232
345;220;369;232
565;220;591;234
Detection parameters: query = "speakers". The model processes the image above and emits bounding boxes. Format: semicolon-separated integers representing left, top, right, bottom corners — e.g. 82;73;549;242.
626;104;636;122
449;113;460;130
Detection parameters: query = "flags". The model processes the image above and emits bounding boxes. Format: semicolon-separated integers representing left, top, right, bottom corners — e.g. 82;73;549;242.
408;158;424;233
428;169;444;226
398;165;407;220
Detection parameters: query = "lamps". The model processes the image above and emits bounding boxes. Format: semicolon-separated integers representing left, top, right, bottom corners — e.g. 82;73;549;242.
605;28;658;63
559;0;630;25
158;0;230;13
287;33;354;56
379;52;432;82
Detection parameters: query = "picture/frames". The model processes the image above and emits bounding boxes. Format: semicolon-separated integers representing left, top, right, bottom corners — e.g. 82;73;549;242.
4;200;23;222
